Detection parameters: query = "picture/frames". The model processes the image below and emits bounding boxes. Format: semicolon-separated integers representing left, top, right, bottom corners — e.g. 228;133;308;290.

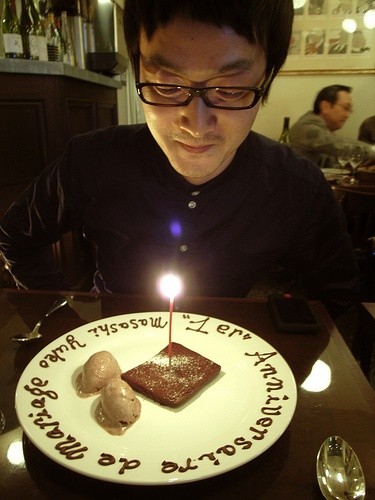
275;0;375;75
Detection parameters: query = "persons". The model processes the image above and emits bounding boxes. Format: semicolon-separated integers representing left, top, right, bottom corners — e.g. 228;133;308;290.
290;85;375;168
0;0;353;389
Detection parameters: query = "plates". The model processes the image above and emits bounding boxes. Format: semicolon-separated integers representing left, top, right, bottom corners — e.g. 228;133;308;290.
14;311;297;485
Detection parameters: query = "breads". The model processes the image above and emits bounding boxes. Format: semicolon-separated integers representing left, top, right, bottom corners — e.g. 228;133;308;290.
120;342;222;409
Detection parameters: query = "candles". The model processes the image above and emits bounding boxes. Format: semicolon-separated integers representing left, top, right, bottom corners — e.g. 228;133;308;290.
168;296;174;357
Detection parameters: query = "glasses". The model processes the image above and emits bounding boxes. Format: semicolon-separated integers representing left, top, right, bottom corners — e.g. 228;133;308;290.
335;102;353;113
132;49;275;110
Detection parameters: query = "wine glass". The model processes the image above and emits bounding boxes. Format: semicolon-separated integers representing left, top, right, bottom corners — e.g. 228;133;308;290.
338;142;364;185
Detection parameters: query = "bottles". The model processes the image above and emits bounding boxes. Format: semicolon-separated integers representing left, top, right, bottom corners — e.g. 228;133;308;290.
278;117;290;147
1;0;97;70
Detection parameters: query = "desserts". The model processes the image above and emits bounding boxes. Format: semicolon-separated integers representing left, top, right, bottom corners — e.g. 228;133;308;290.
82;350;140;428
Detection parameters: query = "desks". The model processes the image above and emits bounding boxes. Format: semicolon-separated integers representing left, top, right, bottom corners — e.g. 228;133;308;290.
0;284;375;500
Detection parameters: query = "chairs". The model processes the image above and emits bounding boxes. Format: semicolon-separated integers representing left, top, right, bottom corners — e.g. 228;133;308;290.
331;186;375;289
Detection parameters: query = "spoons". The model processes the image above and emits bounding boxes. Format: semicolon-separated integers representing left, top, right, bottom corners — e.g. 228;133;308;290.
10;296;72;341
315;434;367;500
